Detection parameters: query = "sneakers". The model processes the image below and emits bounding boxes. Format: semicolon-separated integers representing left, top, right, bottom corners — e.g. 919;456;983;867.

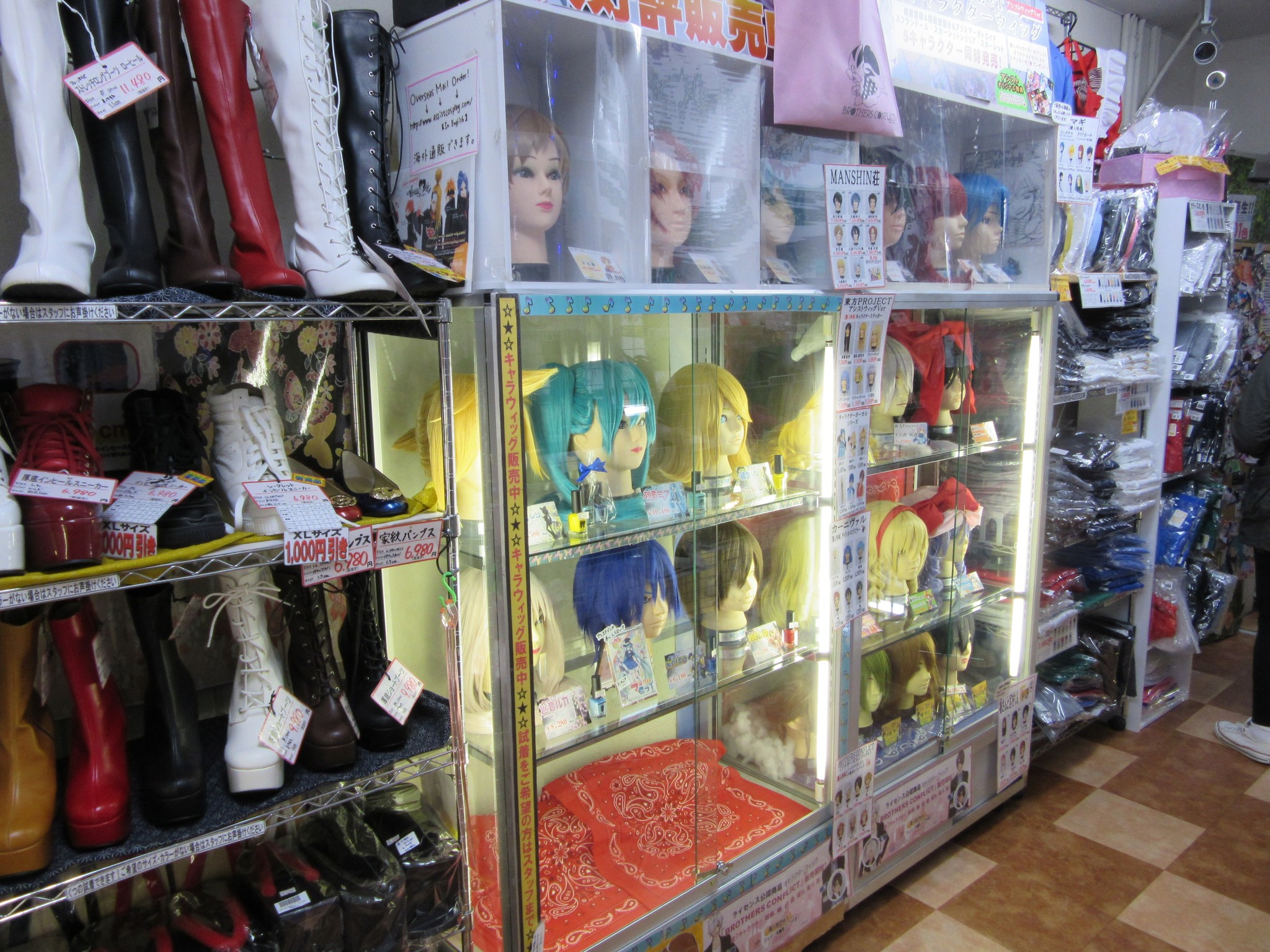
1214;716;1270;764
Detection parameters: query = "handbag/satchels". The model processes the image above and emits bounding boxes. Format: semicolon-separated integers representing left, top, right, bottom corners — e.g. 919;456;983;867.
774;1;907;138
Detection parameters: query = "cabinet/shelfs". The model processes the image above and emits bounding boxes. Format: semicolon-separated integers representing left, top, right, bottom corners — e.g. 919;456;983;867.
1026;269;1159;763
703;291;1060;811
382;0;1060;296
1;293;477;952
453;288;846;950
1114;198;1240;733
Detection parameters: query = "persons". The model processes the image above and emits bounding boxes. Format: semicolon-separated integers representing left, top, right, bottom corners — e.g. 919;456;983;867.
447;568;566;708
672;522;764;646
571;540;674;687
539;361;651;501
417;372;528;514
760;162;811;284
504;101;570;282
1214;342;1270;764
645;362;752;484
644;130;703;282
856;144;1012;748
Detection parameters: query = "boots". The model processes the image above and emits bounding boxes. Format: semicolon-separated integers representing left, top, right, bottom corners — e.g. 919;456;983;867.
0;0;467;952
